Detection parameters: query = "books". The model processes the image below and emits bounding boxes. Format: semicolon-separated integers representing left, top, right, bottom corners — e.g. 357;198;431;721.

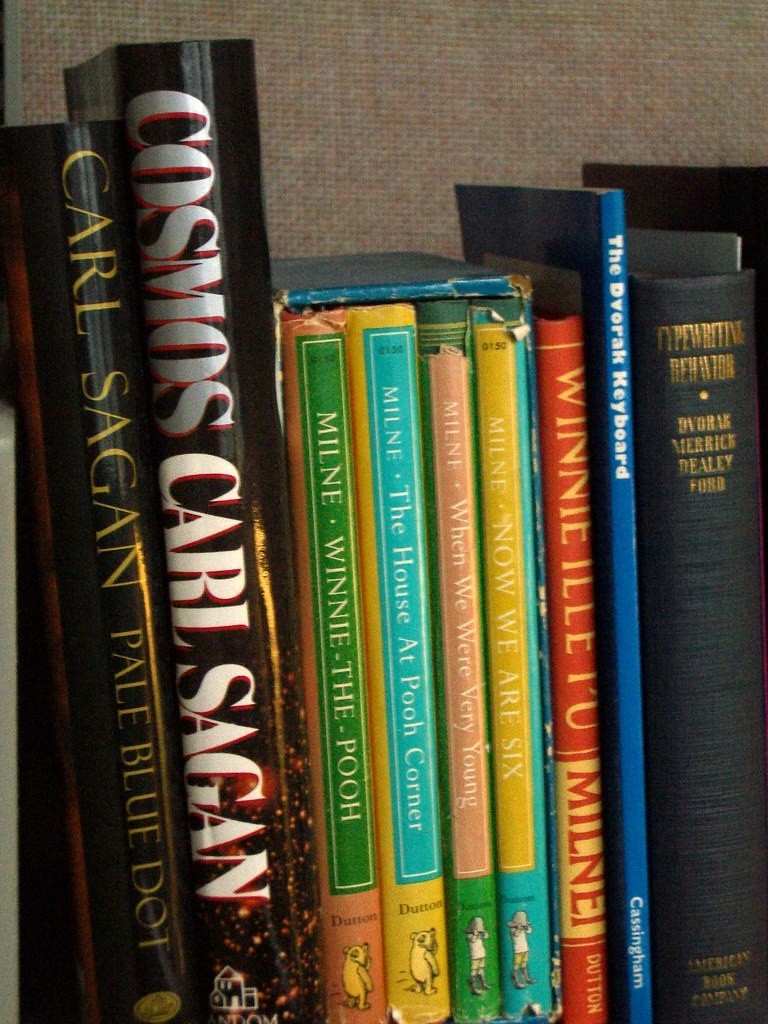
63;39;325;1024
0;121;768;1024
